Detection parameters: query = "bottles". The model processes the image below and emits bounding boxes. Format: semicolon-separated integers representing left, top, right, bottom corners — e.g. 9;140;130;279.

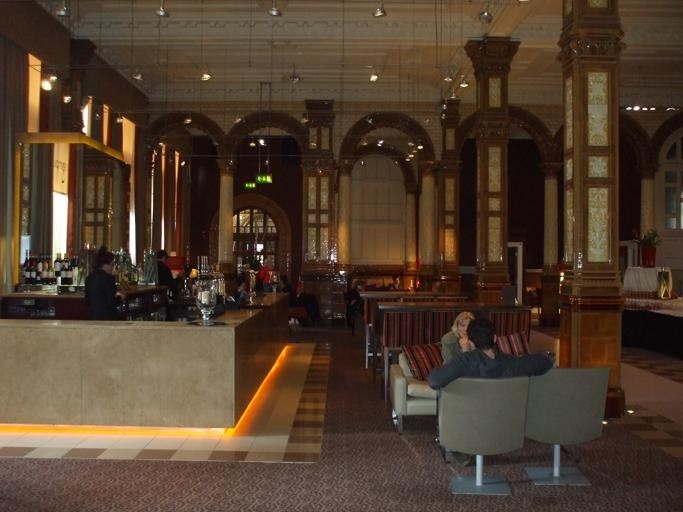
23;250;54;284
216;277;226;295
195;255;210;278
55;252;80;285
656;266;673;300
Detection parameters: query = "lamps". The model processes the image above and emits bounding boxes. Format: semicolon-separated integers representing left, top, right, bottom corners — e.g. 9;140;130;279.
115;113;125;124
257;82;272;185
479;5;493;25
377;139;383;148
55;1;71;17
250;131;264;147
369;73;379;83
132;68;143;81
155;3;169;17
63;95;73;104
440;74;468;120
267;2;282;16
287;66;303;84
41;70;61;91
183;113;194;124
367;118;373;125
181;160;186;165
200;71;211;81
373;3;386;20
405;140;423;165
234;114;246;126
361;142;368;145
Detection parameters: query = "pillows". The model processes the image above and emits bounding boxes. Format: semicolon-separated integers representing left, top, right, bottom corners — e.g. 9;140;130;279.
401;337;443;381
493;332;532;355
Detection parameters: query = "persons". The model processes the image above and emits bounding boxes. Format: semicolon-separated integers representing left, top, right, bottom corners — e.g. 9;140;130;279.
154;249;176;295
229;278;247;304
429;318;553;388
84;247;125;321
442;311;475;364
344;278;363;328
389;273;400;290
258;258;272;293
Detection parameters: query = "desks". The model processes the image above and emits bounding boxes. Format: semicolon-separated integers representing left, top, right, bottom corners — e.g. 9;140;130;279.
624;264;672;292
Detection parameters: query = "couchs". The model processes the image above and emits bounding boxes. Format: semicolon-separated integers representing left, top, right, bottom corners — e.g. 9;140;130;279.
378;331;542;430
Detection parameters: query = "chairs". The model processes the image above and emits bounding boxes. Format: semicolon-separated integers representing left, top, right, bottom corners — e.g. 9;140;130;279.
436;372;529;498
508;363;615;489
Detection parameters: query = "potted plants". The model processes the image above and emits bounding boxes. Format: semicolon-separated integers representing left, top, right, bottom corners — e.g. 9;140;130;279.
637;226;659;267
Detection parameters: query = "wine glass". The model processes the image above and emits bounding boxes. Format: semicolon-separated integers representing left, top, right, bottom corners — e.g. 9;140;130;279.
195;282;218;324
